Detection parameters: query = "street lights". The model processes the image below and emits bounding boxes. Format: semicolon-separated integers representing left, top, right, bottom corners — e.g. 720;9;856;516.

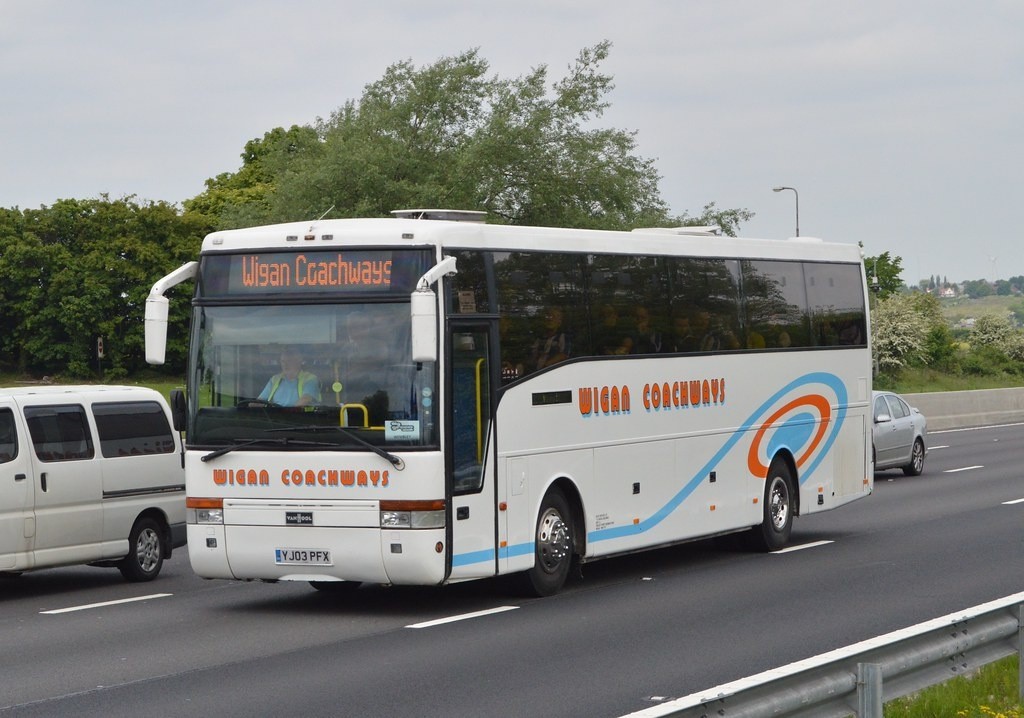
772;186;800;237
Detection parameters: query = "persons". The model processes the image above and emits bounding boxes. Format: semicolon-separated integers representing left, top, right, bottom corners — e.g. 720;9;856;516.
252;309;392;406
495;285;863;383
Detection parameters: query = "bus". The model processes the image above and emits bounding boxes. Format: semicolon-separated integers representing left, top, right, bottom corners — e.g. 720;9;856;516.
143;207;875;600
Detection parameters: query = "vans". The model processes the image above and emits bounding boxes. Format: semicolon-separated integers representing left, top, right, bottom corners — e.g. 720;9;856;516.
0;383;188;583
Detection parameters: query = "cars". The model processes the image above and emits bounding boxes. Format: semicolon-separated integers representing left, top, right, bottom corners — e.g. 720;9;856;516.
871;388;930;475
